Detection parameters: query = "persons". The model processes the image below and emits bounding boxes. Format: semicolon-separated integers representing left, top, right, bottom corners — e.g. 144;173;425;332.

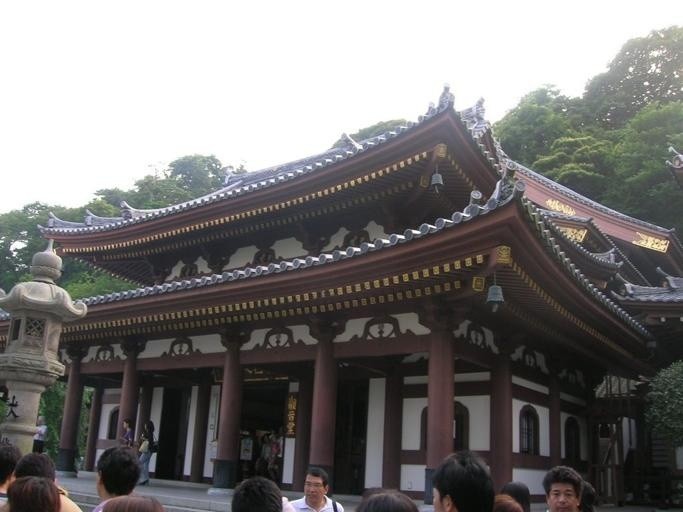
241;434;283;480
357;488;417;512
494;482;530;511
432;451;495;511
543;466;595;511
0;418;165;512
232;477;282;511
288;467;344;511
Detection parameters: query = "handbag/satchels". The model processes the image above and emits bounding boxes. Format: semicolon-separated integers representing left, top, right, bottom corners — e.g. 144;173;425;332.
151;442;160;452
139;440;148;452
